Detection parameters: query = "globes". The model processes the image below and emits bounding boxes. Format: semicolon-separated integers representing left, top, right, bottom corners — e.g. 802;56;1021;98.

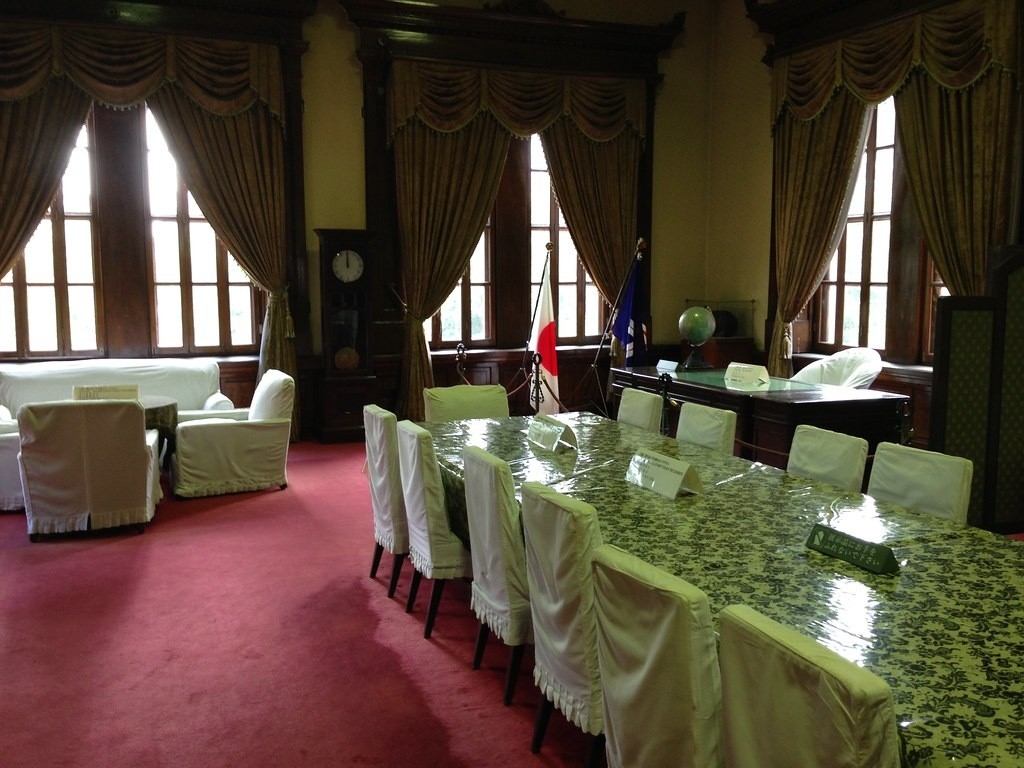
676;304;717;372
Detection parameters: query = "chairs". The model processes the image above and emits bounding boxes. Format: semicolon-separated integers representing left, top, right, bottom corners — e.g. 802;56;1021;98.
422;384;506;420
363;403;413;598
617;388;665;435
395;418;475;637
458;445;536;706
677;402;737;456
590;544;726;768
866;442;972;526
518;483;606;768
717;603;902;768
787;424;868;493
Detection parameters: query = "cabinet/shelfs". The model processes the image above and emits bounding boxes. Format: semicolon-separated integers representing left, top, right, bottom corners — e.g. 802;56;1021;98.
793;350;935;448
679;335;756;369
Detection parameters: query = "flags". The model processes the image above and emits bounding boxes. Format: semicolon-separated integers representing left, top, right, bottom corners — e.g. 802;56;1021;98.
530;259;559;413
611;251;651;369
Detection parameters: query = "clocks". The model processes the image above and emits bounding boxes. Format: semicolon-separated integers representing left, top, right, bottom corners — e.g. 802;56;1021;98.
314;228;378;444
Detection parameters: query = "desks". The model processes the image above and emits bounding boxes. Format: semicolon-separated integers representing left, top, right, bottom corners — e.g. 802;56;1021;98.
409;412;1023;768
625;366;910;468
137;395;179;472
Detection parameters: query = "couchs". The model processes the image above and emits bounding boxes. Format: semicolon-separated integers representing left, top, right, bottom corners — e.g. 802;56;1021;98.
0;357;232;410
173;368;294;499
17;397;162;542
0;405;26;512
